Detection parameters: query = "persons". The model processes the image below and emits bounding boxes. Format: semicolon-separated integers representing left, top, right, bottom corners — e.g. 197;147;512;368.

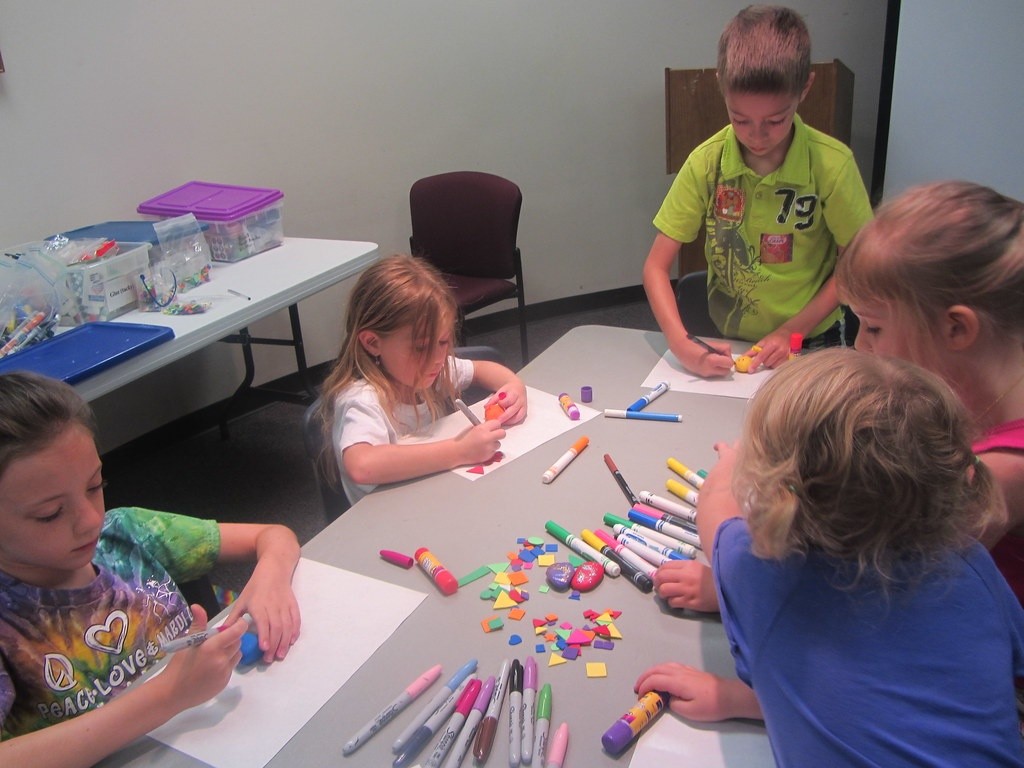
1;370;301;768
318;255;527;509
642;5;876;380
635;349;1024;767
652;179;1023;612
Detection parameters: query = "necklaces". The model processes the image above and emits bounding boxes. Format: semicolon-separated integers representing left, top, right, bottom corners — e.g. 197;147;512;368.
974;376;1022;421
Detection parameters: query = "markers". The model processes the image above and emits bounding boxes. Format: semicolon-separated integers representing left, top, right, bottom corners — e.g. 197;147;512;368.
581;457;710;596
0;308;60;358
455;397;481;426
391;655;570;766
161;625;230;652
627;382;668;411
545;521;621;578
341;664;442;756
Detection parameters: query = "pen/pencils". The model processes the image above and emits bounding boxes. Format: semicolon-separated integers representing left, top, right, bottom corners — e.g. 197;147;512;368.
604;408;683;424
686;333;737;364
603;453;639;508
542;434;589;484
226;288;251;301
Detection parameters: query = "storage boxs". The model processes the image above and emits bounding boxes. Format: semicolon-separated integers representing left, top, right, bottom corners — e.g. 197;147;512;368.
44;221;209;289
138;181;283;263
0;240;153;325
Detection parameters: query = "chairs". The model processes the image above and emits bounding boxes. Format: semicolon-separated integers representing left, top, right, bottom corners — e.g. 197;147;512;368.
407;172;530;364
301;346;506;522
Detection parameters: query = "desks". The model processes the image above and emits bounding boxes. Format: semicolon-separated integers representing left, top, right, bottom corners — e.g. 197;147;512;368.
84;326;811;768
55;239;380;445
